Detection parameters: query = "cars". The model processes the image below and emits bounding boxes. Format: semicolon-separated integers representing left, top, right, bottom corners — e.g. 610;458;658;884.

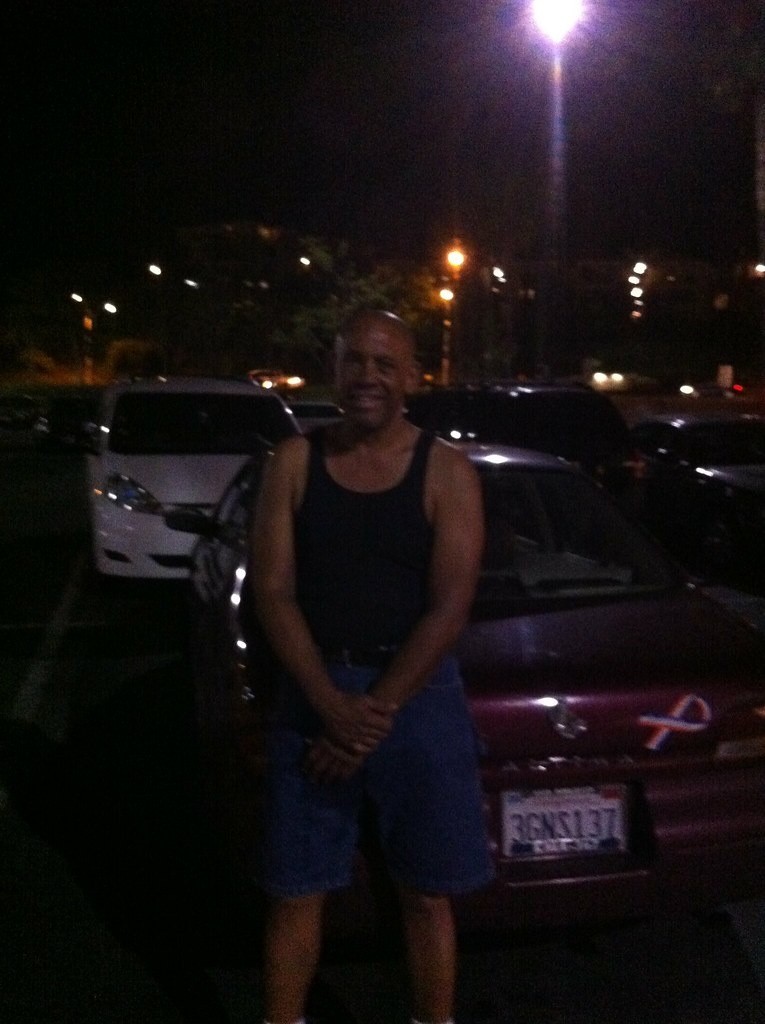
690;383;749;399
245;370;294;392
0;393;50;451
626;412;765;565
166;442;765;930
58;379;304;595
35;395;99;453
462;368;594;396
288;399;346;431
601;369;659;393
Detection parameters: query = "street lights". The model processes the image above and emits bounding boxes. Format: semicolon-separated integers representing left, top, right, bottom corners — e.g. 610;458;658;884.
440;242;466;384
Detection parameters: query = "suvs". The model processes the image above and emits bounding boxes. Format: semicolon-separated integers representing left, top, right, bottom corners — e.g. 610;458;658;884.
402;381;730;562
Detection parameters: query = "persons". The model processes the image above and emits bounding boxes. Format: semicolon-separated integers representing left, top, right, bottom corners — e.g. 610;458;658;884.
248;310;496;1024
